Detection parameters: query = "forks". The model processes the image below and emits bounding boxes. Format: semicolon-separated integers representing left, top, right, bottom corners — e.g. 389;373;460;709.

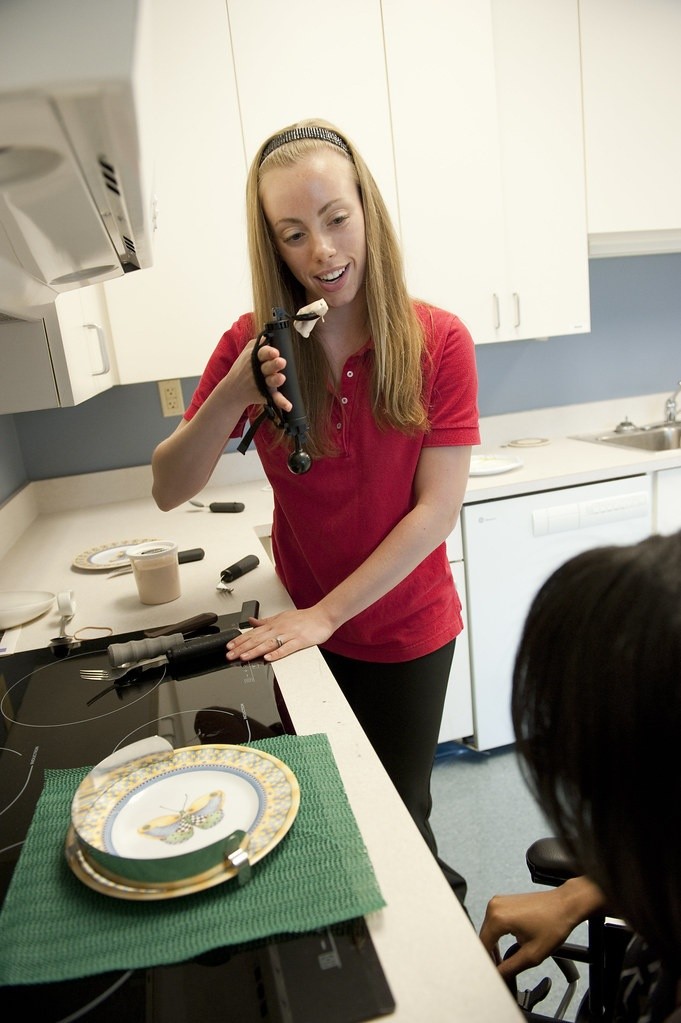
215;550;261;592
76;629;246;681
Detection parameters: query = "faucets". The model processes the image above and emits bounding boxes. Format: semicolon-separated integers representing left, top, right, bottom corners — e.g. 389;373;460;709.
664;379;681;423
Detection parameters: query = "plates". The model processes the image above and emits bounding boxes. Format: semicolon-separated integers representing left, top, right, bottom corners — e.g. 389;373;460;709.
505;435;549;448
72;539;176;570
0;591;55;630
468;451;524;477
63;742;301;904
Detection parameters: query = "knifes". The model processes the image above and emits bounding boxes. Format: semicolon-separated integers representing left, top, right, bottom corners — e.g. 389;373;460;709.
106;548;206;574
107;626;254;668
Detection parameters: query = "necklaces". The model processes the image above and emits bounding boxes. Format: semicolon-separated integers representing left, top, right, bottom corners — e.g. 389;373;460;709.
319;314;366;373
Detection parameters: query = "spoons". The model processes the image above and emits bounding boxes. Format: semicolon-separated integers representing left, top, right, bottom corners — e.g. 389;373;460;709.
187;501;245;512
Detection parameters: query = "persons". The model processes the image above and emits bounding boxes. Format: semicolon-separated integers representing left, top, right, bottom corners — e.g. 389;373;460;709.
479;535;681;1022
151;120;483;930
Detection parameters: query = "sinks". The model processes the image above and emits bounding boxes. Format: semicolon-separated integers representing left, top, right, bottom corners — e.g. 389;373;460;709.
566;419;681;455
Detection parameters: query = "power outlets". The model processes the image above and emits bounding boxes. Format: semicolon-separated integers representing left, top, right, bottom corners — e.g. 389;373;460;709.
157;380;185;417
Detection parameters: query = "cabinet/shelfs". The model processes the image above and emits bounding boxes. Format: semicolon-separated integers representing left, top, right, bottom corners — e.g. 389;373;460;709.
0;0;681;414
260;465;681;755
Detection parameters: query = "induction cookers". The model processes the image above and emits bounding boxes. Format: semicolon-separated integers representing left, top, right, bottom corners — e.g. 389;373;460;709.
0;609;396;1023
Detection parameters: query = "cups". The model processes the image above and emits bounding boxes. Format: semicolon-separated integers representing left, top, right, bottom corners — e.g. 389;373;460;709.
127;540;181;605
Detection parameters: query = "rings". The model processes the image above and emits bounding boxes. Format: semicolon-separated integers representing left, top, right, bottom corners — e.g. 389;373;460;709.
275;637;283;647
